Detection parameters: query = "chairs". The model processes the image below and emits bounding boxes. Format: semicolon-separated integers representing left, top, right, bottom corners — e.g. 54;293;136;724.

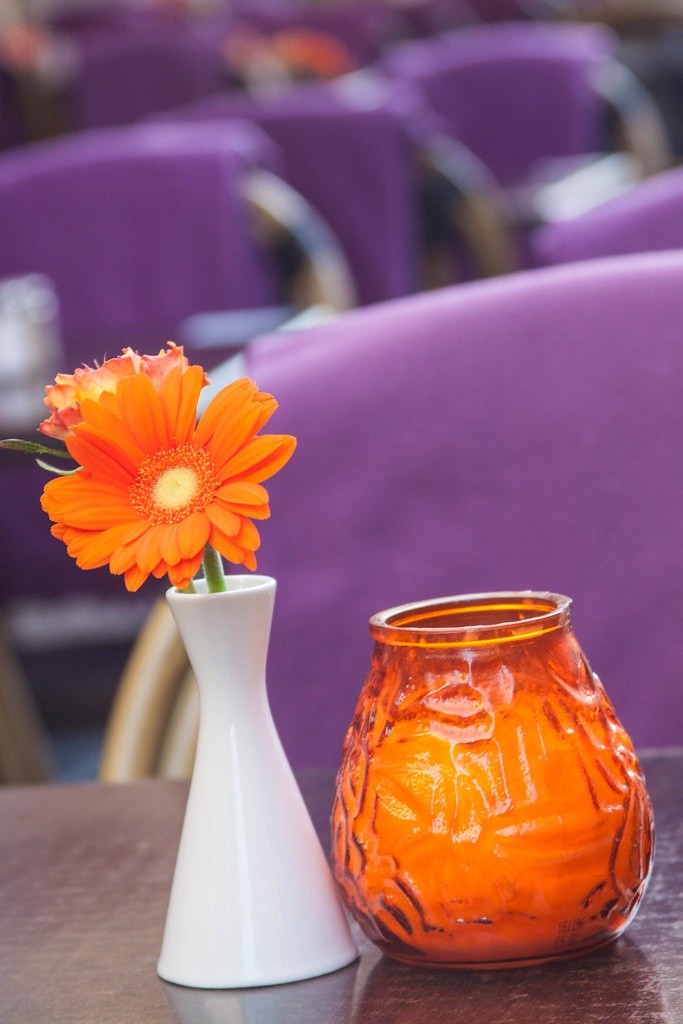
99;249;682;786
0;0;681;252
0;118;358;649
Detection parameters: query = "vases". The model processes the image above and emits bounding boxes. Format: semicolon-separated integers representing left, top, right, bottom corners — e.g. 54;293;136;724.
156;573;359;989
331;591;657;970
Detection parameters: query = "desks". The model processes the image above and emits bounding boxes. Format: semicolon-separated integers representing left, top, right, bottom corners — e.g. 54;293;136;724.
0;748;683;1024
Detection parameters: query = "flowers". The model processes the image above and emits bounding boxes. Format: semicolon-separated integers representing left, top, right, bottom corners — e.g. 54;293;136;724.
0;338;301;591
